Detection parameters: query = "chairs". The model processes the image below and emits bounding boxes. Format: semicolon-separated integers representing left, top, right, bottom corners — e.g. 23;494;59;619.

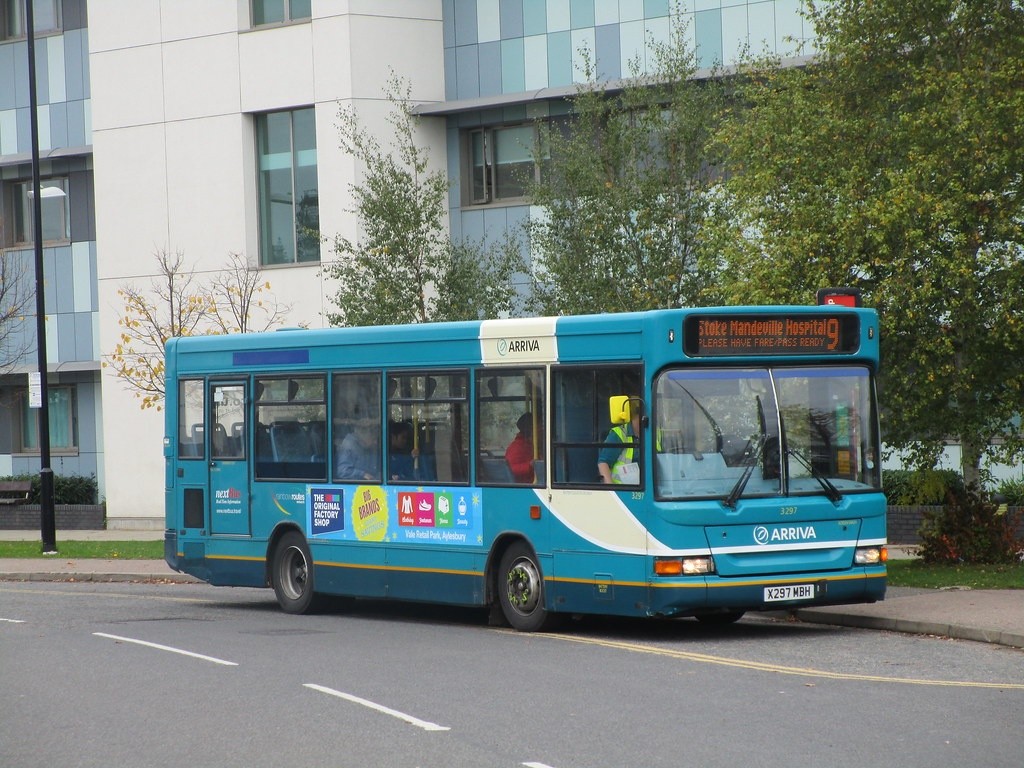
190;420;325;463
408;420;605;481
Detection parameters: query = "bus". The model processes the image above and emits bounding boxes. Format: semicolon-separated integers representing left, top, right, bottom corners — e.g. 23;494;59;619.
166;307;890;633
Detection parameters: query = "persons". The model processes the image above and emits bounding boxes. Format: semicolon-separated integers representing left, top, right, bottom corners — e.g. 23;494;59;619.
389;423;419;481
337;378;397;479
598;415;662;484
505;412;544;483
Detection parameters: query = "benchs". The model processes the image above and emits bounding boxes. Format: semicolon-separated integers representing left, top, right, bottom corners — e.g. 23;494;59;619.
0;479;33;504
